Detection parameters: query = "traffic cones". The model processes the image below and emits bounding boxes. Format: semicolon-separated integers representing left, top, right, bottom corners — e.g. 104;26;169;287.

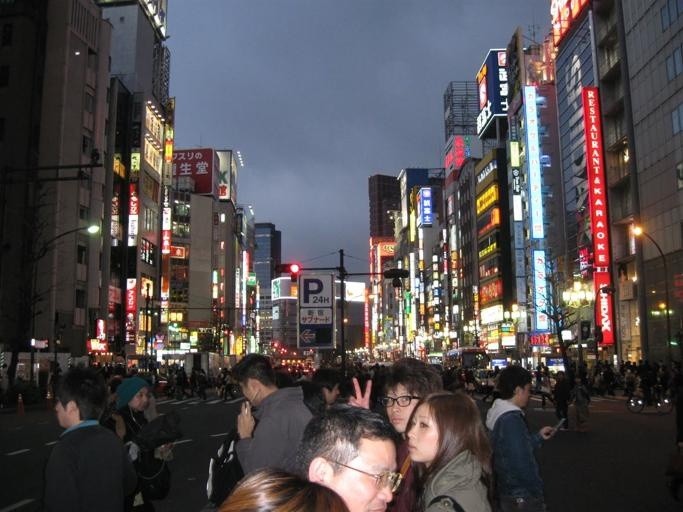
46;384;53;411
17;393;25;418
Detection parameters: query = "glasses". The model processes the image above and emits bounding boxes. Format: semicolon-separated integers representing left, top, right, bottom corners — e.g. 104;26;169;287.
382;395;419;408
327;458;402;493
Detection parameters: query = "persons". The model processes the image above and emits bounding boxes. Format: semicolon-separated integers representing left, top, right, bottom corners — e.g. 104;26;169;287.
229;355;317;480
42;368;138;512
101;377;175;512
298;405;401;512
485;366;555;511
347;356;442;512
213;466;348;512
448;365;475;395
405;391;493;511
148;367;235;402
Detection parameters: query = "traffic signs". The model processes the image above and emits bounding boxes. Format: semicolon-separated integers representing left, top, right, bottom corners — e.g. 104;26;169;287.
384;268;409;278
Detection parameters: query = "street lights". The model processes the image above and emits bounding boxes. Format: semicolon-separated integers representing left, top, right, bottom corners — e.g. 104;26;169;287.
29;224;100;384
631;226;673;363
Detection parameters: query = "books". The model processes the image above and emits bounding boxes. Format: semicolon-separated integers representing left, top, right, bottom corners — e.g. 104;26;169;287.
536;358;683;432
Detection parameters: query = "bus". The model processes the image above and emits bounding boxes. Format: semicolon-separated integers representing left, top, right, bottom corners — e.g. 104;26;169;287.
444;346;489;391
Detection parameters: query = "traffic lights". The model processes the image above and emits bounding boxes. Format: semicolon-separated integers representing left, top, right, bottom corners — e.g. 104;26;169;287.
581;320;591;340
278;262;301;275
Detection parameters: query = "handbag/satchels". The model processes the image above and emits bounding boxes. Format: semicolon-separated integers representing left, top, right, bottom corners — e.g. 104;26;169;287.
203;426;244;502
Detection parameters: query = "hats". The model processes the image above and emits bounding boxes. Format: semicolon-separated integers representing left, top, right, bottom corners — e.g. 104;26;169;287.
115;378;148;412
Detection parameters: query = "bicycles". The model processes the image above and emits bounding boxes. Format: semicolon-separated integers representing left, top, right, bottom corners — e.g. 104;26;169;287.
625;383;674;415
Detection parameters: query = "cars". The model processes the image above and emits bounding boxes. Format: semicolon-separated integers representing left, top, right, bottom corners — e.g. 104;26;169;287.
473;368;496;390
